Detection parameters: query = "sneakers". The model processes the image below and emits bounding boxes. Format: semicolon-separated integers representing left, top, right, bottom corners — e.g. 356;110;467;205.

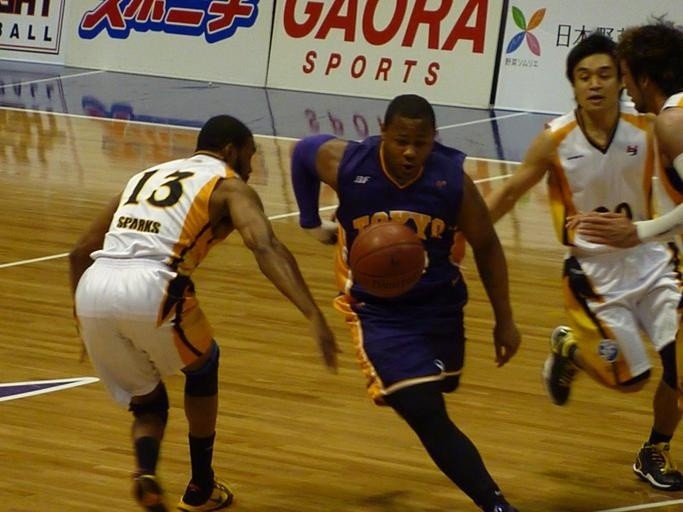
631;438;683;491
541;325;579;405
178;476;233;512
129;474;168;512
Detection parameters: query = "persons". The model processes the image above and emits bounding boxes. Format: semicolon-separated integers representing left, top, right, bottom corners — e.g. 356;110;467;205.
560;24;682;249
66;113;343;511
480;29;682;491
289;93;523;512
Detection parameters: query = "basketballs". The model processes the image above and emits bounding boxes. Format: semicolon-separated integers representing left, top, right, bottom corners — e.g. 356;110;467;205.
349;220;425;297
